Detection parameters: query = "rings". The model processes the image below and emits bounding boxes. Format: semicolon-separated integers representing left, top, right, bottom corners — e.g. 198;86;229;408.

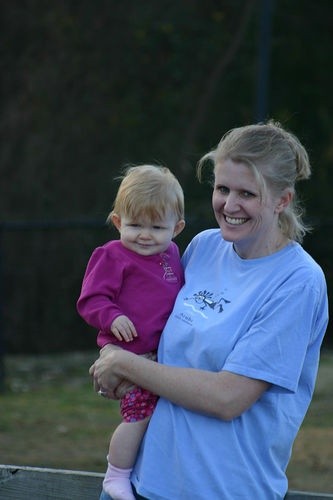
97;390;105;397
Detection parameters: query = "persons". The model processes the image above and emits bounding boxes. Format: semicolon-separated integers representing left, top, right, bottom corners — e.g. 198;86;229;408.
89;124;329;500
76;164;186;500
